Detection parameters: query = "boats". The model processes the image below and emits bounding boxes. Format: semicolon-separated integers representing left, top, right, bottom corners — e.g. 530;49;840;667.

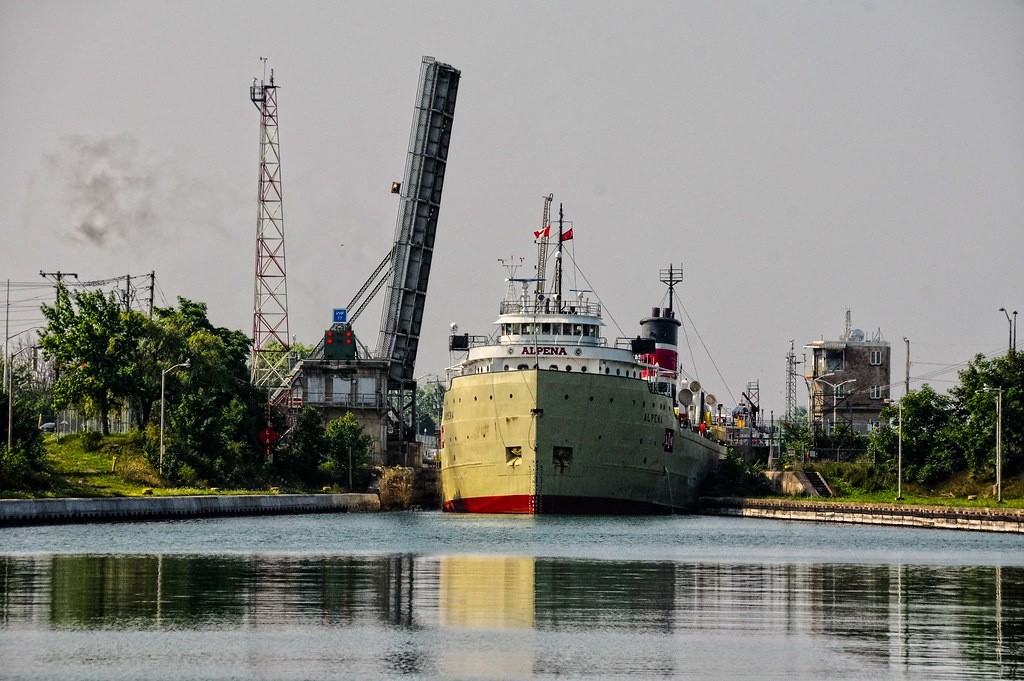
439;193;728;514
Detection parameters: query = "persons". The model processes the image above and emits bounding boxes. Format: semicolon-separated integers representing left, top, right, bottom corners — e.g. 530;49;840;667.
700;420;707;437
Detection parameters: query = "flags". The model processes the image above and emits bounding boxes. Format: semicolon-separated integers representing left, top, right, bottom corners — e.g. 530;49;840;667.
562;228;573;240
534;225;550;238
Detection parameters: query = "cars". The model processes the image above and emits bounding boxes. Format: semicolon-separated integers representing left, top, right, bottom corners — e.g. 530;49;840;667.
285;397;302;408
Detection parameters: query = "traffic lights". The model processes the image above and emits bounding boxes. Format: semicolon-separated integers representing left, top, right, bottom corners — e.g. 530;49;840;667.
324;330;355;360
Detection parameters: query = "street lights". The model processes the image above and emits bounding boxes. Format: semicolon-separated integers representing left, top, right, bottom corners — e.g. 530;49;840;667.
159;363;191;475
975;383;1001;502
1013;310;1018;349
884;399;905;500
796;373;835;430
3;325;47;394
816;379;857;432
8;345;44;452
999;308;1012;354
